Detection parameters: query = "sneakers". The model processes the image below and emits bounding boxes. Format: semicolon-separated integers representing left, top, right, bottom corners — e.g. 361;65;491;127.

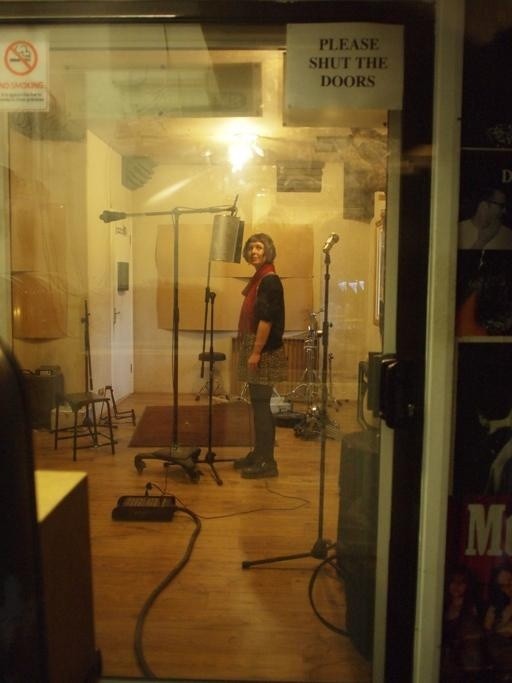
233;452;278;479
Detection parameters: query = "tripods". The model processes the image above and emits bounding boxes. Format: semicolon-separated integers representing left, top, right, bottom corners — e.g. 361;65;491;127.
52;303;116;448
241;253;349;578
160;264;241;485
133;215;203;484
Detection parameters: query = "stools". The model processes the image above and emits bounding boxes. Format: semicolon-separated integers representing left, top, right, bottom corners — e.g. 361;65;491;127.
52;392;116;461
194;352;229;402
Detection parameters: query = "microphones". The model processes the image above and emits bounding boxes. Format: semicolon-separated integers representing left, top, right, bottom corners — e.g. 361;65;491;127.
322;233;338;253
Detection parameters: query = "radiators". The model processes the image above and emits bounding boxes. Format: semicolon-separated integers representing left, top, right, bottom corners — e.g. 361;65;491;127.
229;337;320;401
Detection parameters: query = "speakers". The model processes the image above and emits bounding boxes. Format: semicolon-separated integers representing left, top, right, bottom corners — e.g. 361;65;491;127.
208;214;241;266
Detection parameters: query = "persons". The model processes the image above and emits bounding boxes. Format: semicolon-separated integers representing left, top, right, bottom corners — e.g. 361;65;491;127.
456;186;512;253
230;232;290;479
447;567;480;627
478;564;512;638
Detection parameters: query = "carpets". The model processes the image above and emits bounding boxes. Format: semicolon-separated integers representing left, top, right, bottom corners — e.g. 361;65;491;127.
127;402;256;449
